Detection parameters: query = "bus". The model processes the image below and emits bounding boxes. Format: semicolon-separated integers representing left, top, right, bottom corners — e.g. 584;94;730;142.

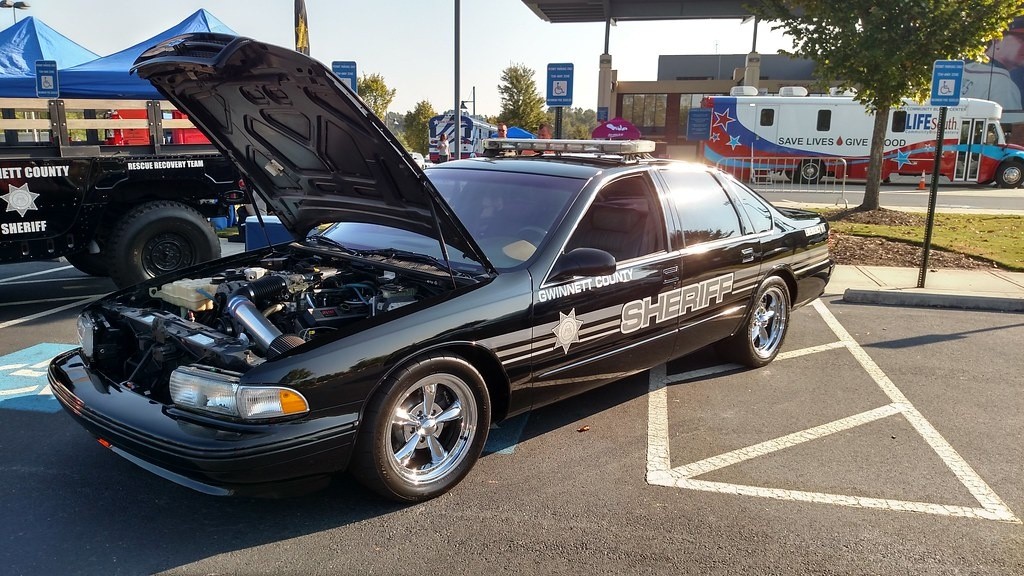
697;85;1024;190
429;114;499;164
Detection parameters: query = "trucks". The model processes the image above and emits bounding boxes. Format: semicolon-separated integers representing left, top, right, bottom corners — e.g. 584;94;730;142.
0;95;257;294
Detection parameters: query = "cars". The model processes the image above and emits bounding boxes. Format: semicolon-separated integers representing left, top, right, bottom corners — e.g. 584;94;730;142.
46;31;832;503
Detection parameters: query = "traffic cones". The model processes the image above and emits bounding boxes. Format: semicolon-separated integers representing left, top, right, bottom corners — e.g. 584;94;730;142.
917;169;927;192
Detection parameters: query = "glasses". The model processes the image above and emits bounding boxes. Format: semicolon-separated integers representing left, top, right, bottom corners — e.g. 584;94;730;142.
498;129;505;132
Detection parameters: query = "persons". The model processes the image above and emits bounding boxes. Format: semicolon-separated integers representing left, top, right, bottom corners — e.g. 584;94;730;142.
482;122;517;157
520;124;556;157
437;133;452;163
